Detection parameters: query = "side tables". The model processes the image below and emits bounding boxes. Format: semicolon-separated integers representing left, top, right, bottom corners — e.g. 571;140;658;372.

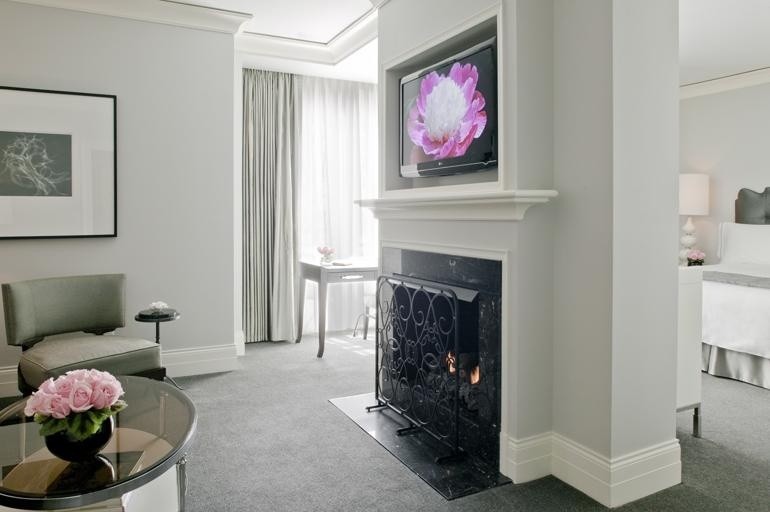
135;308;185;391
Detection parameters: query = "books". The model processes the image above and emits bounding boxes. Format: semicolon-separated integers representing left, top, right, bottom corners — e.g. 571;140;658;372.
333;260;352;267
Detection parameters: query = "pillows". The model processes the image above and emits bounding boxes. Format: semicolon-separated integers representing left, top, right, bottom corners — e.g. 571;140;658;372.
718;221;770;266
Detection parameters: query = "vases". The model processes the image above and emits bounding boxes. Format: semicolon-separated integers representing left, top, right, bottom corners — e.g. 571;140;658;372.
688;262;703;266
320;256;334;265
44;412;114;461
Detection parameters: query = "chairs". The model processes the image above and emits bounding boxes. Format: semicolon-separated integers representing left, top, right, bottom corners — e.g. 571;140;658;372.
2;272;167;400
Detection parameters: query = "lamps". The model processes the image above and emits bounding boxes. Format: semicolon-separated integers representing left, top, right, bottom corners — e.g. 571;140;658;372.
678;172;710;266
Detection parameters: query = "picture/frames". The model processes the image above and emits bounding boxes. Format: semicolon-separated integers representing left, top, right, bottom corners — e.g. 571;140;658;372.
1;85;118;239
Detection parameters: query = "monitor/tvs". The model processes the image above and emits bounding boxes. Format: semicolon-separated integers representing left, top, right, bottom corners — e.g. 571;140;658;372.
397;37;496;176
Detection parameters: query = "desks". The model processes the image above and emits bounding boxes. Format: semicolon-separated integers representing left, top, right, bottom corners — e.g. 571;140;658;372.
294;258;377;358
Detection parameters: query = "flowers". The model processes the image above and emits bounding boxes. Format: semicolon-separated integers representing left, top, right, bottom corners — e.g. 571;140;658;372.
687;251;706;263
406;62;488;164
23;367;129;443
317;246;336;255
